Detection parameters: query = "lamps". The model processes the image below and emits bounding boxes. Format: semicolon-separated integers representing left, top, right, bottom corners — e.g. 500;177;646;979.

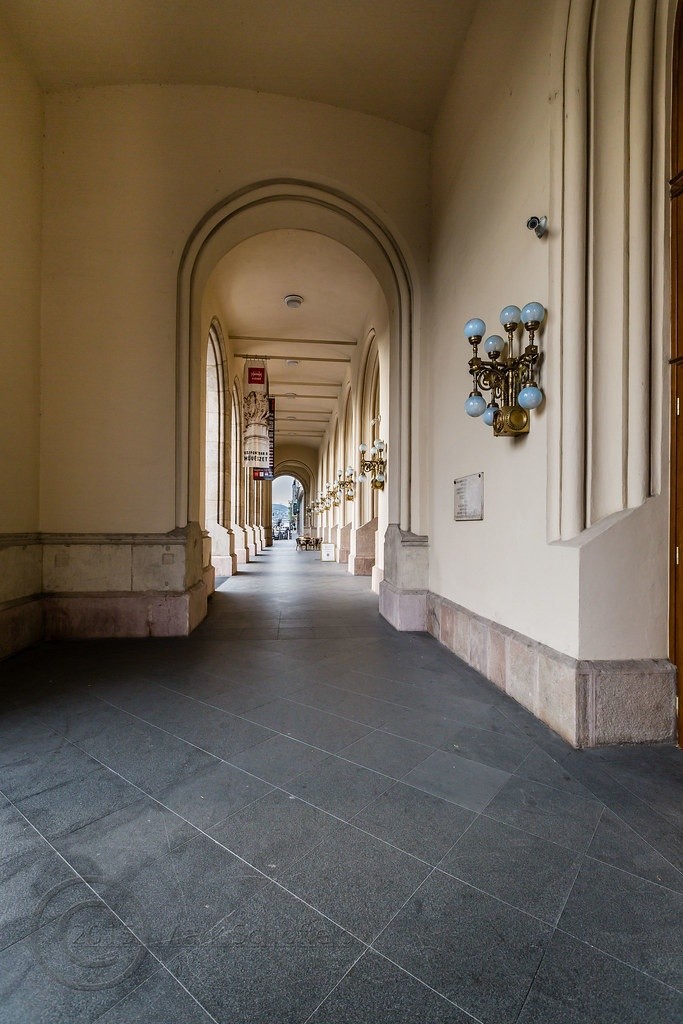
305;438;387;517
284;295;304;309
286;360;299;367
463;302;544;437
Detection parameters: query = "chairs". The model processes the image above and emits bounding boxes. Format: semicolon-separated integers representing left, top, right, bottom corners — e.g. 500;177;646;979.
296;536;322;551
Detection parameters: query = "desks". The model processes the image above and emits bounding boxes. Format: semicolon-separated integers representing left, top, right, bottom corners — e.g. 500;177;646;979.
300;539;313;551
